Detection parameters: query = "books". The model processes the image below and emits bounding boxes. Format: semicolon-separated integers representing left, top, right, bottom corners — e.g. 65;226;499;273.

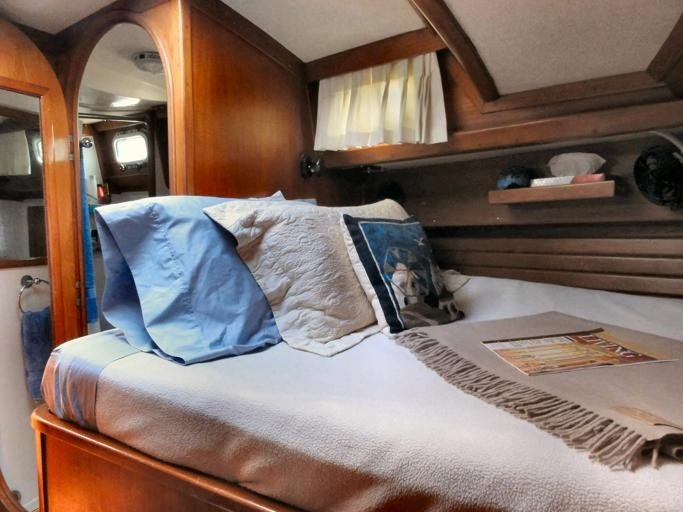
480;328;680;377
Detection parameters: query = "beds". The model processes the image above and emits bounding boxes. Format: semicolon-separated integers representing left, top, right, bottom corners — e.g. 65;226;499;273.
32;274;682;512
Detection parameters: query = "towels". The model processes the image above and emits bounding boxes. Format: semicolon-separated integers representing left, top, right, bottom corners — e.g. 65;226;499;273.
20;304;52;403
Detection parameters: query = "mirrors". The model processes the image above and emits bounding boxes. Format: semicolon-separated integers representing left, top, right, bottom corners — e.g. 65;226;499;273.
0;89;48;269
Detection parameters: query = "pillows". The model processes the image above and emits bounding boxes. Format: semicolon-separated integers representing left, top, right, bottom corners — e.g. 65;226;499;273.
203;199;468;358
340;215;466;335
93;190;282;367
